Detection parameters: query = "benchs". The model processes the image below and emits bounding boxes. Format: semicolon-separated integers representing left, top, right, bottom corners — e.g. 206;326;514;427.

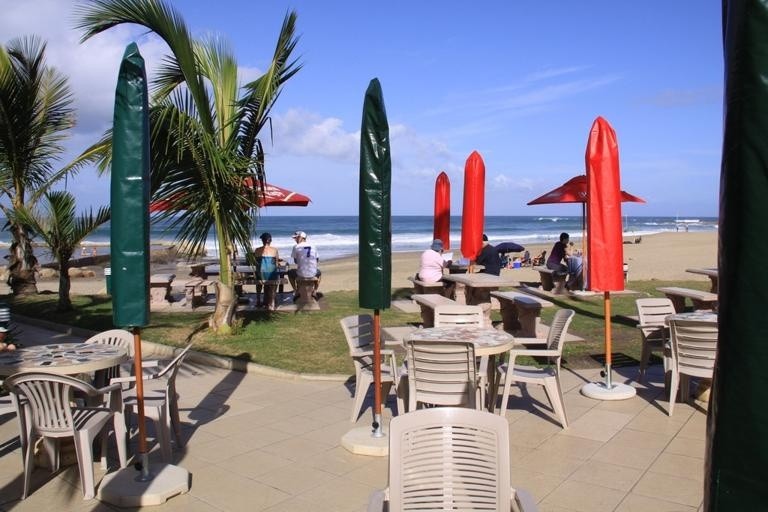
149;258;320;312
491;291;554;337
655;286;719;314
407;276;446;294
534;265;580;294
413;294;456;328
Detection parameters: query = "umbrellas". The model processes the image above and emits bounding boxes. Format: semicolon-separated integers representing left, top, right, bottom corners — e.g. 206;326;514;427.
495;242;525;270
96;43;191;508
432;171;450;253
149;176;310;304
703;2;767;510
579;116;639;401
527;174;647;297
339;77;391;458
461;151;486;273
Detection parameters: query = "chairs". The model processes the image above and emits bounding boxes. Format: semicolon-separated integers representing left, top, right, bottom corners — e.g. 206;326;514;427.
488;309;573;429
109;339;195;462
434;305;494;406
407;338;486;410
668;316;718;416
359;409;531;512
3;372;128;498
85;331;134;418
637;298;678;396
339;313;409;420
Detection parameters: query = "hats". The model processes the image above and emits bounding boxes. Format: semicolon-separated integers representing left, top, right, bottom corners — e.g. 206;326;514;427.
292;231;306;239
431;239;443;251
482;235;488;240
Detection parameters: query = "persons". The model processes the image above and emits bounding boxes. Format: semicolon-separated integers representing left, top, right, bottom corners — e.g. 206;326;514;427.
499;242;580;270
474;234;500;276
254;233;279;307
675;222;690;233
287;231;323;302
80;246;97;257
418;239;455;298
0;326;17;352
546;232;571;294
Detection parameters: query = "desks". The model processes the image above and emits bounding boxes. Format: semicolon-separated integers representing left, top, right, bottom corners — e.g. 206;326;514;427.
687;268;723;296
6;344;129;470
443;274;520;328
408;327;513;416
